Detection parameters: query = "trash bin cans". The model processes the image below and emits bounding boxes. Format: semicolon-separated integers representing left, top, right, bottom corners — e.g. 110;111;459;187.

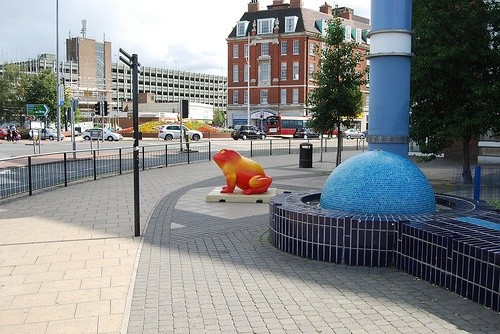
298;143;314;168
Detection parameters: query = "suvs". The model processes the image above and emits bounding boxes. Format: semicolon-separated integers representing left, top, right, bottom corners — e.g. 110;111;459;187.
231;124;267;141
158;124;203;141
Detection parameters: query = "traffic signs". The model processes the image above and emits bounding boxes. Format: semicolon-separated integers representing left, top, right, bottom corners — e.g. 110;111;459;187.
26;104;50;117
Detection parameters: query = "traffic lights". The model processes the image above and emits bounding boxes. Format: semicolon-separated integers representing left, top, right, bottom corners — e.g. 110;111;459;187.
104;101;110;116
95;101;101;116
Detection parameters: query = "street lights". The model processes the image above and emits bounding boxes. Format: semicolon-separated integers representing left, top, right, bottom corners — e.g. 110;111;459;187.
245;32;262;125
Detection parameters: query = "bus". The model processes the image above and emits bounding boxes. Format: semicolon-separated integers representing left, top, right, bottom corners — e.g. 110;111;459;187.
265;115;320;139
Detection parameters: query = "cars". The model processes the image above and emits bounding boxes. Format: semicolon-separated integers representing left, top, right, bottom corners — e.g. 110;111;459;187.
0;127;21;141
323;128;346;138
345;128;366;140
38;128;65;141
82;128;124;141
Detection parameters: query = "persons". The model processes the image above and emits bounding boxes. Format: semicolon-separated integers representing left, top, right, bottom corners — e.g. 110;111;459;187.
6;126;11;142
11;124;17;141
